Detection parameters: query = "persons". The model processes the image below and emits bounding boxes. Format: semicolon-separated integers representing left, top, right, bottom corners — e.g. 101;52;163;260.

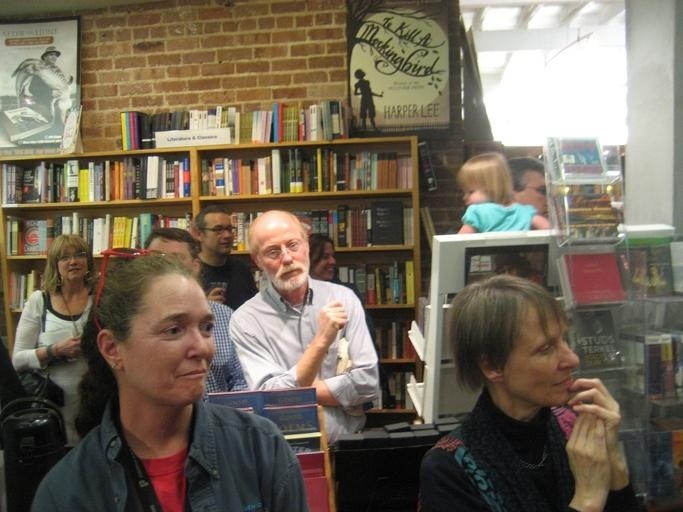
32;247;310;510
11;233;98;445
9;46;74;128
351;69;384;128
506;158;551;231
453;151;549;235
417;275;647;510
228;209;381;444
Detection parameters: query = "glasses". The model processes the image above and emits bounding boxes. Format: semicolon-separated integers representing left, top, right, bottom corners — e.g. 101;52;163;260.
199;225;238;234
56;251;87;263
524;185;547;196
259;241;299;259
92;247;168;332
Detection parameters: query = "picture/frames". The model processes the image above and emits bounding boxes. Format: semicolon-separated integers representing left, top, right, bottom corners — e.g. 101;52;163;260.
0;14;81;156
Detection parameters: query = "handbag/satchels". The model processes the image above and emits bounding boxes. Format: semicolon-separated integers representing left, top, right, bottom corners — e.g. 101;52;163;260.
15;289;65;409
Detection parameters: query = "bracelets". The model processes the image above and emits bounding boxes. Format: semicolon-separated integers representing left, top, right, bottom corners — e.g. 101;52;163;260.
45;343;58;365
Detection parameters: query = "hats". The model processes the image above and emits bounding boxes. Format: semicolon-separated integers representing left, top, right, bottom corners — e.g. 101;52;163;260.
41;46;61;60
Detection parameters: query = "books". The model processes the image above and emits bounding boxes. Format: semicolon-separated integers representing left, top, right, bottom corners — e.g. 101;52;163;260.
201;146;412;194
11;270;45;311
227;201;414;247
208;386;333;511
255;261;414;305
418;140;438;192
545;138;682;510
6;213;191;257
374;320;416;411
420;206;436;253
120;99;348;151
2;156;190;204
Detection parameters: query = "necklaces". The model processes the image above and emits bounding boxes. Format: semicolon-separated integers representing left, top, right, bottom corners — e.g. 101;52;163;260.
517;443;547;471
58;288;85;336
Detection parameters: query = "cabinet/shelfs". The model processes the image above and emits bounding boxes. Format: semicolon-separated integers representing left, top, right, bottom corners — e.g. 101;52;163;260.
407;223;683;425
0;135;419;420
